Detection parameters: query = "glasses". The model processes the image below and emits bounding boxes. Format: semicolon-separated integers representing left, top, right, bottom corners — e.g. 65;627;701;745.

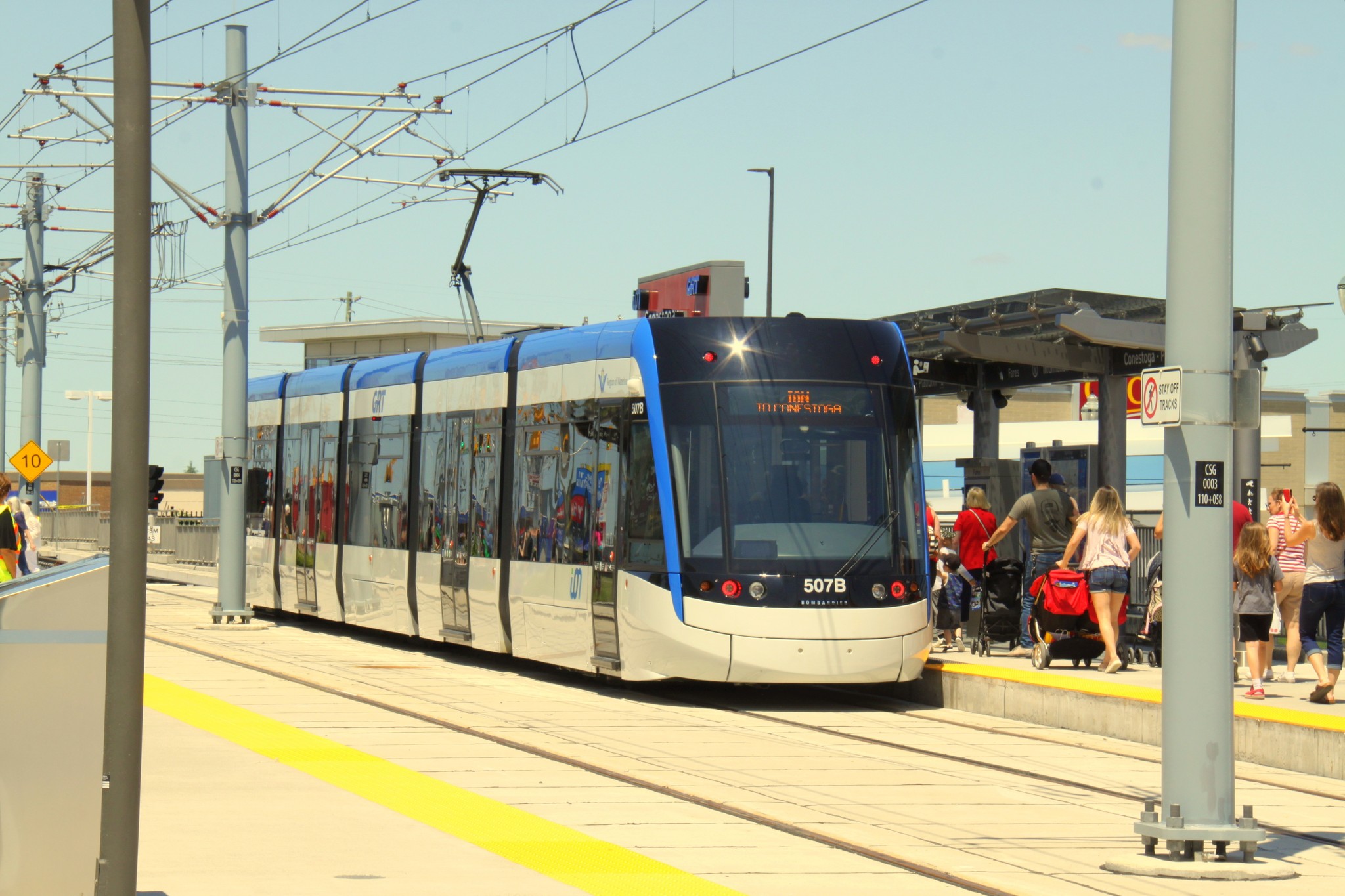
1313;495;1316;501
1265;500;1279;507
26;503;31;505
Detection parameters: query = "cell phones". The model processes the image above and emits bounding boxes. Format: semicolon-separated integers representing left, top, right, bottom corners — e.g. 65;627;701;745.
1283;489;1293;503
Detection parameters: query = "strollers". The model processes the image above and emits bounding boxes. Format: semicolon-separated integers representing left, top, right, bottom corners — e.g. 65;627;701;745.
1129;550;1164;667
1026;561;1131;671
969;547;1029;657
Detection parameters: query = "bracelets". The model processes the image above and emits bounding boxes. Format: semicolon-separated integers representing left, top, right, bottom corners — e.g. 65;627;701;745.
1284;517;1290;519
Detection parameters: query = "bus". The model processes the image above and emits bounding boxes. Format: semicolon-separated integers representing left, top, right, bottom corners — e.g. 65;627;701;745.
248;176;940;688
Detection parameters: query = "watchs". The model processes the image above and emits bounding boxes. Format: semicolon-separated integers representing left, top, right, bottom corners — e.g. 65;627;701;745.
985;541;992;548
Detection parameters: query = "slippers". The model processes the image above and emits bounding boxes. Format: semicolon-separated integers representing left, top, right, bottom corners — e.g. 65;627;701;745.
1319;697;1335;703
1309;681;1333;702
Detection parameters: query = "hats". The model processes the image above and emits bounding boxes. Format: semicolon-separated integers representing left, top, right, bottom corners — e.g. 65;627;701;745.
1029;459;1052;479
21;498;32;504
944;553;961;570
1049;473;1066;486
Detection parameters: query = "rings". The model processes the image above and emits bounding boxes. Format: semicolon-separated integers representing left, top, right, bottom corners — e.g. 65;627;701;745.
35;548;36;549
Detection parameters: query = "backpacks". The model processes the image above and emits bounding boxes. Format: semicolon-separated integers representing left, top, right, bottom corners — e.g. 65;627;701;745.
1148;578;1162;622
927;525;938;556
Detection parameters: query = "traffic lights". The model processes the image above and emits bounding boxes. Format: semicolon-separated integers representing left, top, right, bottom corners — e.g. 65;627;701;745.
149;464;165;509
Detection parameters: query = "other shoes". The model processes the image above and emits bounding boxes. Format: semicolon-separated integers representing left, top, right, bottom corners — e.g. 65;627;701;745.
1234;657;1239;682
1138;629;1151;641
1007;645;1033;658
1098;663;1109;671
933;637;947;648
955;636;965;651
1245;685;1265;699
932;627;944;637
1277;670;1296;683
1105;656;1122;674
942;645;954;652
1246;669;1274;679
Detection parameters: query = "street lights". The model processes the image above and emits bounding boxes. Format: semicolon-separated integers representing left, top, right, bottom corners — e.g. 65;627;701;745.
65;388;112;511
745;165;774;318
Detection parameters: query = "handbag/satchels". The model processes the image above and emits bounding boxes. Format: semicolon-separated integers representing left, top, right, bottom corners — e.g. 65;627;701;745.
1268;589;1282;634
1044;627;1105;643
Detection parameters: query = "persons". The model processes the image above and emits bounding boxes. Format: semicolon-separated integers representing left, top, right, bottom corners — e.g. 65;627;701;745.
932;530;977;647
981;459;1080;657
1048;474;1079;514
935;552;966;652
1244;487;1308;684
1282;482;1345;705
282;494;293;536
1232;521;1284;699
0;471;43;584
1055;486;1142;674
951;486;999;639
1232;500;1254;683
913;481;944;622
1153;504;1164;539
